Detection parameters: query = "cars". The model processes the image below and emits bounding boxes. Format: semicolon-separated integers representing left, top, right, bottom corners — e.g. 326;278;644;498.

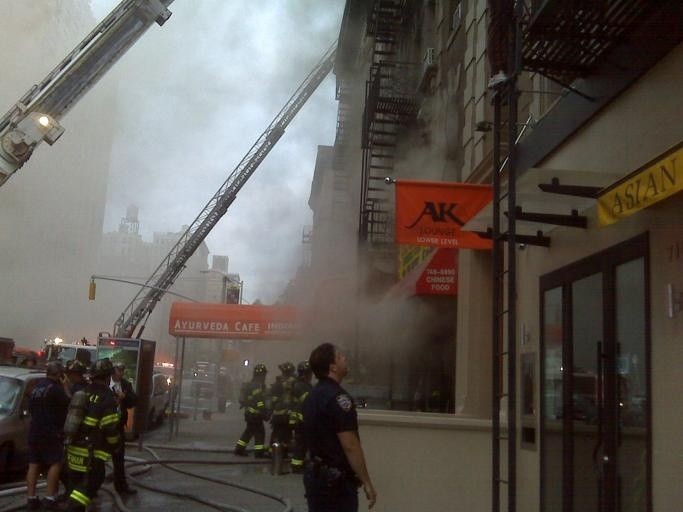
148;360;243;427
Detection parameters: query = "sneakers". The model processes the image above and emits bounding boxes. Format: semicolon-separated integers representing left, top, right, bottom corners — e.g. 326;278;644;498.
234;449;248;456
256;450;269;456
293;465;305;473
27;497;59;511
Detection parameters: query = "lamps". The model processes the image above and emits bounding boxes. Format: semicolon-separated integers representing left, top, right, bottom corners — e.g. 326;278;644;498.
665;281;683;318
473;70;577;136
519;321;529;345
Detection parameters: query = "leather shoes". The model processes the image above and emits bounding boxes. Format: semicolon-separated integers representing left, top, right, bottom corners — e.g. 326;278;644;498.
115;485;139;496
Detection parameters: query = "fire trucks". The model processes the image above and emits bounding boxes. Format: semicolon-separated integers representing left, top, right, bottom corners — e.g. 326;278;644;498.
550;366;598;424
44;37;337;448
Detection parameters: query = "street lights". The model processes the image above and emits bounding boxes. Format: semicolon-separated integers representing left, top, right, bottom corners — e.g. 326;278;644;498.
200;268;244;306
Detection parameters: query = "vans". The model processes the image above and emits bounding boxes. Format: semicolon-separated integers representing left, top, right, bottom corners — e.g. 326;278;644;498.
0;365;47;478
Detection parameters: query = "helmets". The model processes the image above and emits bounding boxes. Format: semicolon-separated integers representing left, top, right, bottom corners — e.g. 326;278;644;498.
254;360;313;376
90;358;115;377
66;360;87;372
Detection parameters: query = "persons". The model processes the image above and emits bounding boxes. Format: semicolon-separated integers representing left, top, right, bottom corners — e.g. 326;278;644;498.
298;343;376;512
7;349;138;512
288;360;314;473
269;361;297;458
235;364;272;457
